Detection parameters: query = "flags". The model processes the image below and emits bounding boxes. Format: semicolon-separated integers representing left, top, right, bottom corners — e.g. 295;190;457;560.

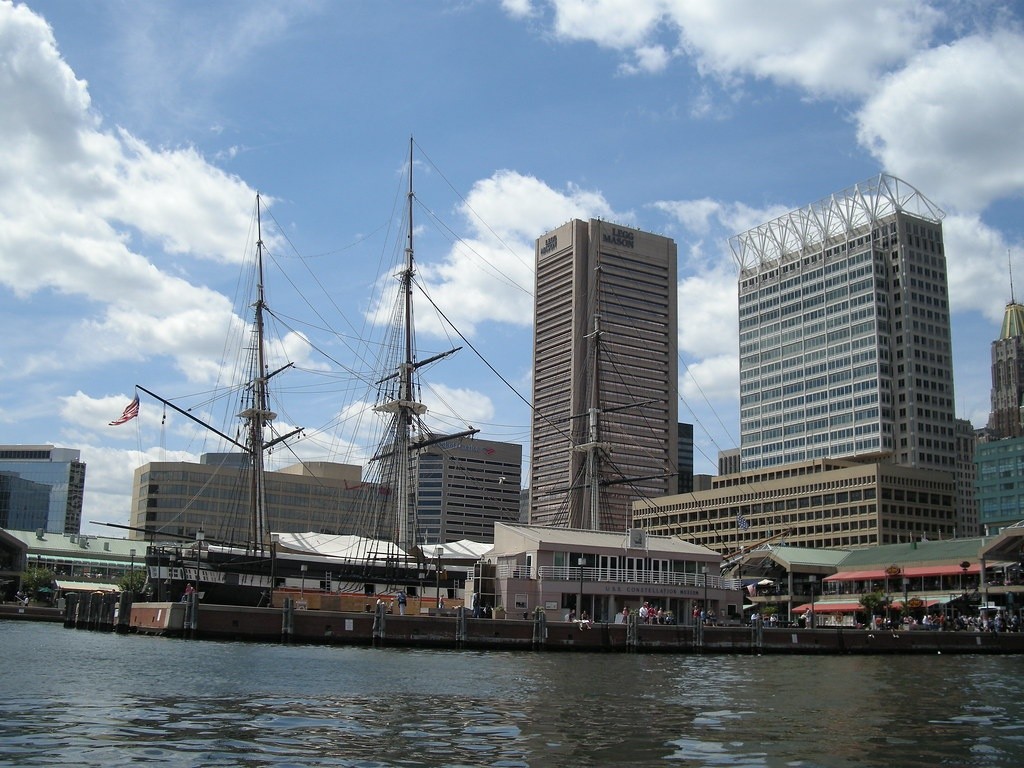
738;513;749;531
747;583;757;596
109;392;140;426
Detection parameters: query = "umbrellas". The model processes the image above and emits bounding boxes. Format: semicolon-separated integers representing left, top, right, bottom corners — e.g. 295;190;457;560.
38;586;55;592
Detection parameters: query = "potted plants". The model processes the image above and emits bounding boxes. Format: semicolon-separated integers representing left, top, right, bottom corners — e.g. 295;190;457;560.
663;610;677;625
532;605;546;620
492;606;507;619
629;609;644;624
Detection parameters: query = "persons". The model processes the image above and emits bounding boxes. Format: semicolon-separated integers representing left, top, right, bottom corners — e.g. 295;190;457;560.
0;588;29;606
184;583;194;601
750;607;812;630
569;602;725;631
847;577;1024;631
377;590;493;618
